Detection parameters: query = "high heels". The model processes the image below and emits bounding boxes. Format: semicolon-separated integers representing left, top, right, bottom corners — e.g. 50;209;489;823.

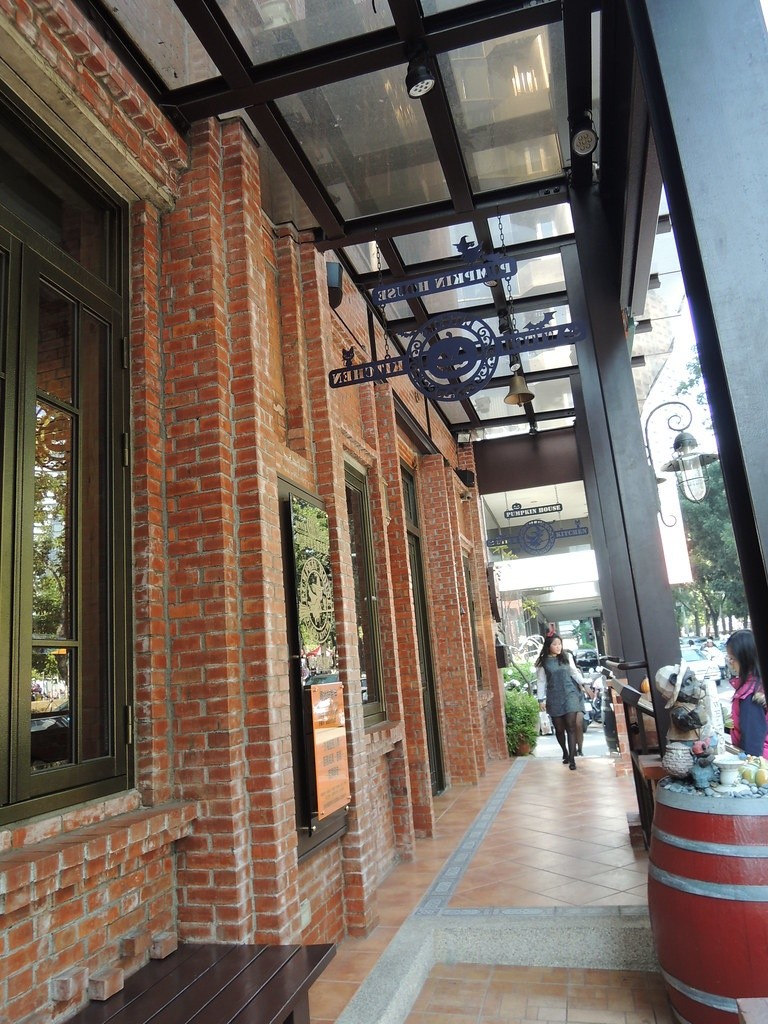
562;752;568;763
568;755;576;770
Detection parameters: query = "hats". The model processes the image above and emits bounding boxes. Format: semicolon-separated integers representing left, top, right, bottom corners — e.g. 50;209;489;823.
655;658;687;709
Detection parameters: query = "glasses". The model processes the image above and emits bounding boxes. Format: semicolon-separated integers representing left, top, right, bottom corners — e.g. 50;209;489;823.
725;657;737;664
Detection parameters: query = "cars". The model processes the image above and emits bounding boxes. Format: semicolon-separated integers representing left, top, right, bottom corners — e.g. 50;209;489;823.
678;632;737;687
576;650;599;671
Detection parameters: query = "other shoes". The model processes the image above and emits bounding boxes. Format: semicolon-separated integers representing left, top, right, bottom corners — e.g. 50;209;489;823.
578;751;583;756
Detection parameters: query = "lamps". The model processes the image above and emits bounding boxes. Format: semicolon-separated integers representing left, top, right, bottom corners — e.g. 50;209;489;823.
484;272;498;288
510;353;521;372
567;108;599;158
644;402;719;528
405;38;436;99
457;433;472;450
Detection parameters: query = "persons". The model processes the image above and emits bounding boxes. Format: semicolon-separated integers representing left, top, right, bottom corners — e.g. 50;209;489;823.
534;631;593;771
688;640;696;648
703;638;724;667
724;629;768;761
561;647;589;758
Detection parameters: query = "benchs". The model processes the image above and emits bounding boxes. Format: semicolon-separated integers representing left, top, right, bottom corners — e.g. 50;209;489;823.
63;936;338;1024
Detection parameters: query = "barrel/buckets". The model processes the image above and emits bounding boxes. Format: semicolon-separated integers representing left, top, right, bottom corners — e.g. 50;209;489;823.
646;784;768;1024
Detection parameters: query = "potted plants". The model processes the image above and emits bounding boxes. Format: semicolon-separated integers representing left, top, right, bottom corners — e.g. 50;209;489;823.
505;688;540;756
